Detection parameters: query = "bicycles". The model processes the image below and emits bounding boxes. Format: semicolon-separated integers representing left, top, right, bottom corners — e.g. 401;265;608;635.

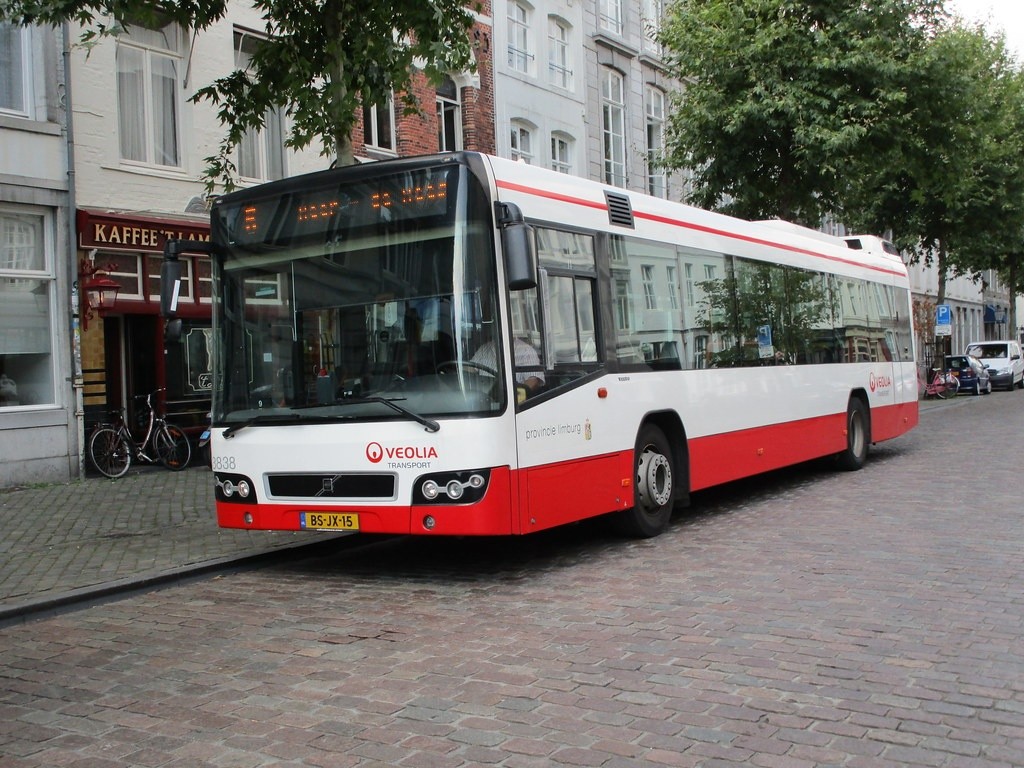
86;387;192;478
919;367;960;399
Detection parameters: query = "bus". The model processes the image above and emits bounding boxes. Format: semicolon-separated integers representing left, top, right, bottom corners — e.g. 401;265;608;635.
155;149;919;533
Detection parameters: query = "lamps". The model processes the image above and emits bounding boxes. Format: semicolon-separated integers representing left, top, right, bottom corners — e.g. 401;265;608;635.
79;258;121;332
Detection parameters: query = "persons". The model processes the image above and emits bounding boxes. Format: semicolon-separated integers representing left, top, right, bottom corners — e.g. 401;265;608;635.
461;335;546;390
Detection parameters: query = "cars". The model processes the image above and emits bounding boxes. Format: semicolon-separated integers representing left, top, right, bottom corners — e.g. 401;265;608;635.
965;340;1024;391
945;355;993;395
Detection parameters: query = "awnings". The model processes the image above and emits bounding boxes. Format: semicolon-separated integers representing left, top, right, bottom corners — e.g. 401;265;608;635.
984;305;1007;324
